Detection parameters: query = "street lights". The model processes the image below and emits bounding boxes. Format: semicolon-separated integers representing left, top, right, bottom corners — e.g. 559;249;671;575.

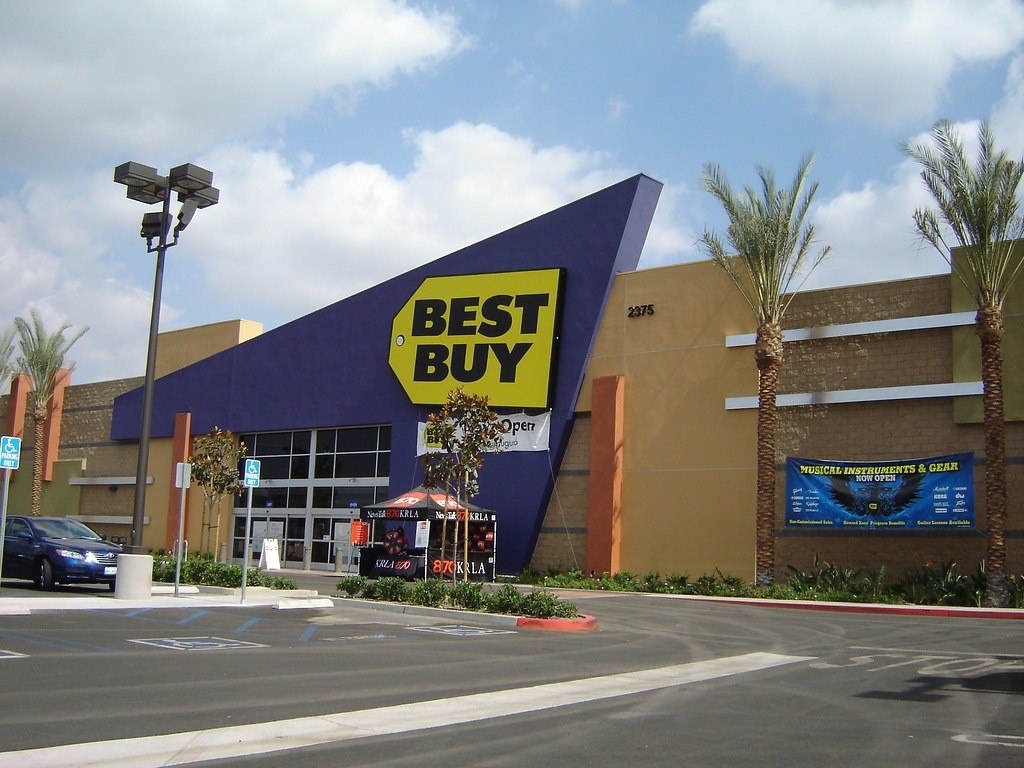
113;159;220;555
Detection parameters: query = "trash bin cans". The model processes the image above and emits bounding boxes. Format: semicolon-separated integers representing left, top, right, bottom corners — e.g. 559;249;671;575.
111;536;128;546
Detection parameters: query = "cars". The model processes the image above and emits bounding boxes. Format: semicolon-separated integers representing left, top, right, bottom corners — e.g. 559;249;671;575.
1;514;122;592
